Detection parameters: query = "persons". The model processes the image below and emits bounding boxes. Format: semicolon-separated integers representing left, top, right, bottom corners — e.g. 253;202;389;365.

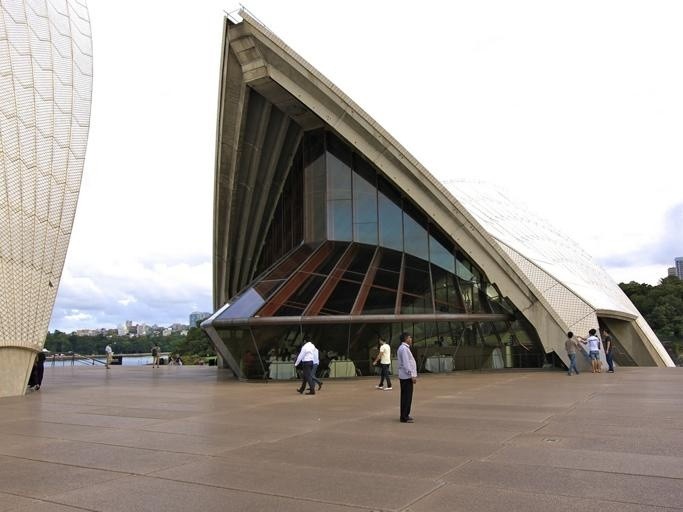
602;327;614;373
396;331;418;423
149;343;160;368
371;335;392;391
240;343;349;379
579;329;600;374
292;333;315;395
27;354;39;388
577;328;600;372
32;350;45;391
565;331;579;376
305;339;323;391
104;342;113;369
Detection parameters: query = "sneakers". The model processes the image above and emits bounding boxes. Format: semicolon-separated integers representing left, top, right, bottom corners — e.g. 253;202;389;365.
376;385;384;389
384;386;394;390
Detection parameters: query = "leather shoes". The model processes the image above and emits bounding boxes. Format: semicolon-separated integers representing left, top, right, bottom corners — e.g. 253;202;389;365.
317;380;323;391
400;416;415;423
306;390;316;395
296;387;302;394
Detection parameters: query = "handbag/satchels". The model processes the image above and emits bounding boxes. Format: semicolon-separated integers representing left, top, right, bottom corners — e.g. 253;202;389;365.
151;348;158;357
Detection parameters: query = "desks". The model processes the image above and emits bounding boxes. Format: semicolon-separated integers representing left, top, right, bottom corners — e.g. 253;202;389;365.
424;356;455;373
268;360;298;380
328;359;357;378
